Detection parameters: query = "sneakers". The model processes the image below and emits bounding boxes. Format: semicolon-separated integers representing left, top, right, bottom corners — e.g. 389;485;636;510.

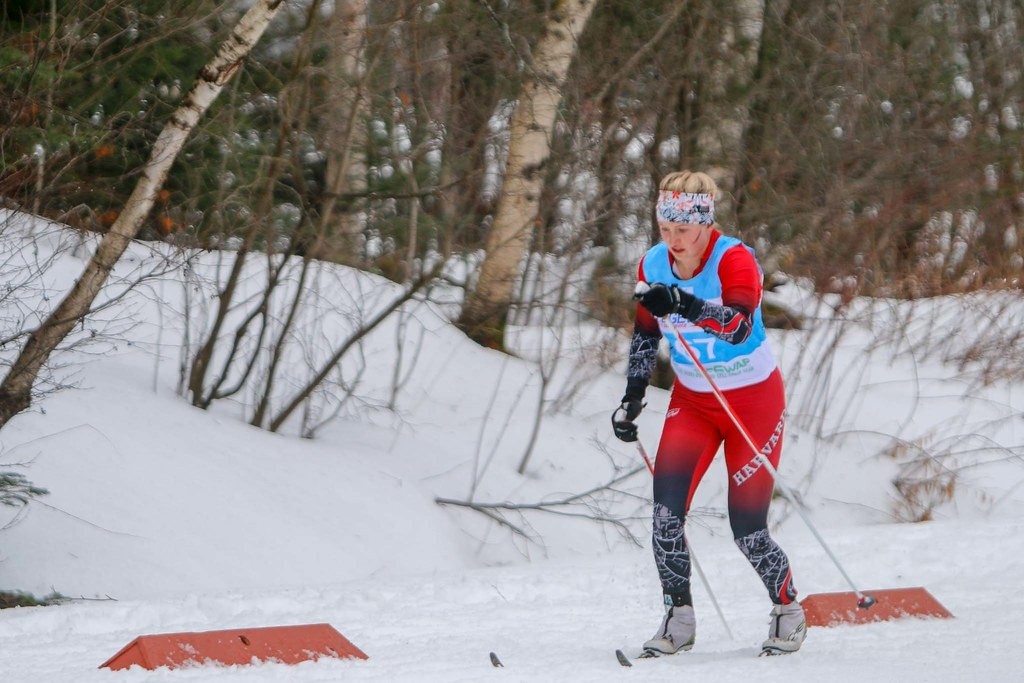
758;600;807;659
643;605;696;655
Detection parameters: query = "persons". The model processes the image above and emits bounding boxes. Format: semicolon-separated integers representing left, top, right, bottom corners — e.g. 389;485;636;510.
612;170;807;656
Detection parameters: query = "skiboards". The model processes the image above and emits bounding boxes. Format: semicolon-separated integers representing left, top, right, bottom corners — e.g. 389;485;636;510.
489;649;634;667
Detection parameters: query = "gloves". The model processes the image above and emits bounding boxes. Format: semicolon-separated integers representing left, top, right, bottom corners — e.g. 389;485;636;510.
612;394;641;441
632;282;691;316
